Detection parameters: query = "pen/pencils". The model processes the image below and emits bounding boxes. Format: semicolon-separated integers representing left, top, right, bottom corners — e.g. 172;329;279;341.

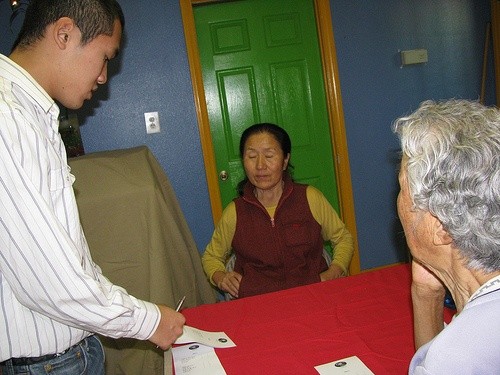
156;296;186;348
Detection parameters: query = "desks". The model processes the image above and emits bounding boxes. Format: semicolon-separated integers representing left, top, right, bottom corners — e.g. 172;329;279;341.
163;262;457;374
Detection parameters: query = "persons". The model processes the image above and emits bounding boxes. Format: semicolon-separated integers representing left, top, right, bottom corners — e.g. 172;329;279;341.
0;0;186;374
392;98;500;375
201;123;354;301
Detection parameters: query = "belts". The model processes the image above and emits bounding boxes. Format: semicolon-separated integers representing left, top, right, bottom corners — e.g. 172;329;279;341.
0;334;94;366
